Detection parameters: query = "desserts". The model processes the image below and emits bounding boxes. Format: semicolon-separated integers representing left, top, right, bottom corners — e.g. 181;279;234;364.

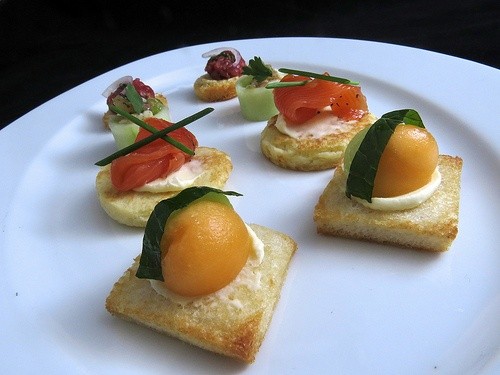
236;55;281;121
103;75;168;128
105;83;153;149
260;68;371;172
195;46;249;102
105;185;297;363
94;103;232;228
312;108;462;251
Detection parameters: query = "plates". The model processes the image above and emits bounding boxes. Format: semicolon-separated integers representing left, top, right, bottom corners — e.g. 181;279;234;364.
1;36;499;375
